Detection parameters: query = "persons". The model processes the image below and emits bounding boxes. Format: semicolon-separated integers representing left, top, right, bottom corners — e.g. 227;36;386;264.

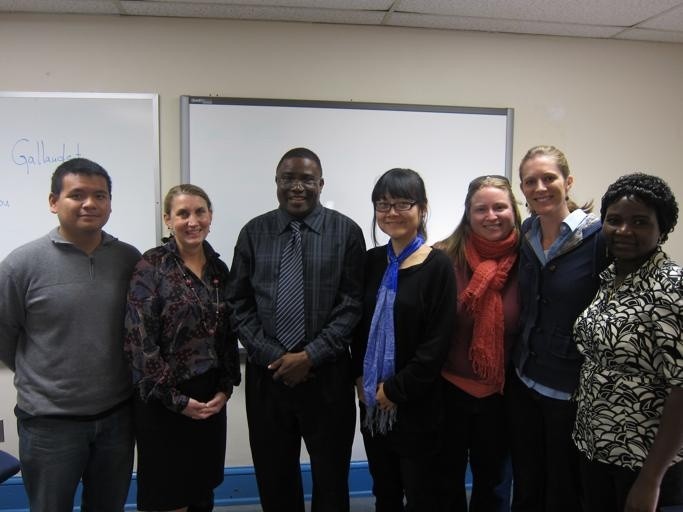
352;163;461;512
0;155;143;510
569;169;683;510
224;144;368;512
429;174;523;512
125;182;242;512
501;139;614;509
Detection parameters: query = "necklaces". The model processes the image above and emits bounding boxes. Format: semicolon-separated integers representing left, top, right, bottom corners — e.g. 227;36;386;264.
172;258;219;341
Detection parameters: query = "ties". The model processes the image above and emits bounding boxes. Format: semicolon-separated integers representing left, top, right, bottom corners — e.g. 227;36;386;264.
275;220;306;352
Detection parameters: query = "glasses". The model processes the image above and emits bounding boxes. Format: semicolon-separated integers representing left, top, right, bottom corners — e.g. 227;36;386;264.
374;201;418;212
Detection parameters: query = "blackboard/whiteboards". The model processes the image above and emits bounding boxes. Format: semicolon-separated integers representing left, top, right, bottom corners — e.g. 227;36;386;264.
184;95;515;357
2;90;162;260
